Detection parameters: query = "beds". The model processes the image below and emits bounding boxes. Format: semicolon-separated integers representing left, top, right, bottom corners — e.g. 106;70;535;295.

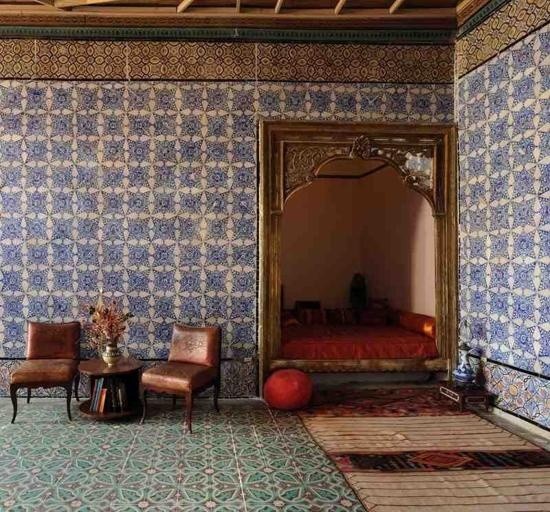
260;120;460;386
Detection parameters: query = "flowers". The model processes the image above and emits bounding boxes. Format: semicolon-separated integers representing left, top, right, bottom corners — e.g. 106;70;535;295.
79;298;136;348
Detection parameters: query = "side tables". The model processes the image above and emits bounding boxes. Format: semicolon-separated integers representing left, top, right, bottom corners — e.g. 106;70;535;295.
78;358;144;422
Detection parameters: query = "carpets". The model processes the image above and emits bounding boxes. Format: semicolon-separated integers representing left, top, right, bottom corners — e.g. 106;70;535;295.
0;393;369;512
297;386;550;511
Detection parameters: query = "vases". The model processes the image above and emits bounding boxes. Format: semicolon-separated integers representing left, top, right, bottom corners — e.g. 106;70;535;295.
102;346;121;367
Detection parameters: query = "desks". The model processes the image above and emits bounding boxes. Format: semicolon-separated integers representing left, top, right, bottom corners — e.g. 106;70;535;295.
437;379;491;413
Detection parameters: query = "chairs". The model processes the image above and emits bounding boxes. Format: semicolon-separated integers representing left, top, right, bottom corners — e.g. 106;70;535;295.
138;323;222;434
9;322;82;424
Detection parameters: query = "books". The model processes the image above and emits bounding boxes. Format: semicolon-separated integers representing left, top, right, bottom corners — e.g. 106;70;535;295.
89;377;108;413
